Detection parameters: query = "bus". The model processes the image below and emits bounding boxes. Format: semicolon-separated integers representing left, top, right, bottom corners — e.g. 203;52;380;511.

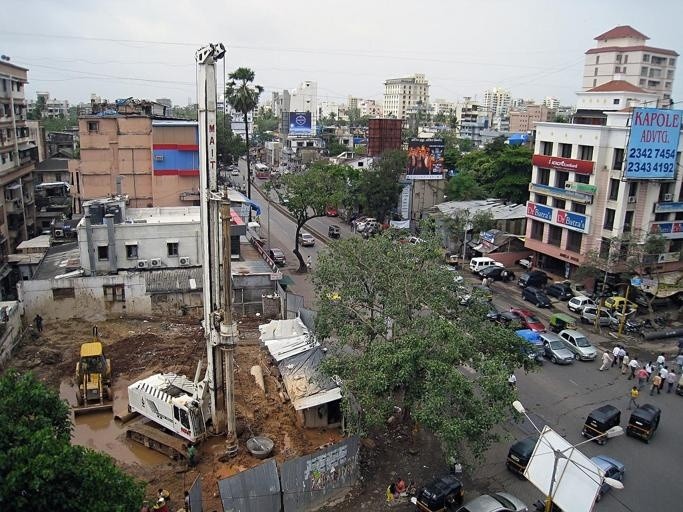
255;163;269;178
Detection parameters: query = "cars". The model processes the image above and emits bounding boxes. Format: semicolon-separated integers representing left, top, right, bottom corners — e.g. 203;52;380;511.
327;205;337;217
675;374;683;396
217;160;240;176
345;213;378;239
447;256;638;365
282;194;289;206
456;490;528;512
268;248;286;267
298;230;315;247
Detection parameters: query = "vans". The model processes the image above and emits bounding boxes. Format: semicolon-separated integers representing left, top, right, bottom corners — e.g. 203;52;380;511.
590;454;626;503
408;474;465;512
506;434;540;480
581;403;661;445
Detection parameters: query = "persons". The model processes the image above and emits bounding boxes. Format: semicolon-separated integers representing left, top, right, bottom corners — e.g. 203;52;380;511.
181;490;190;511
385;482;399;502
394;478;405;493
598;339;682;411
407;145;430;174
482;274;488;287
186;442;196;472
507;371;516;389
306;255;312;273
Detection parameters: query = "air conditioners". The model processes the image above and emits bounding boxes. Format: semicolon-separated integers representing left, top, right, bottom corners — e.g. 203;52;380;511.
662;193;672;201
151;258;162;267
178;256;190;266
628;196;635;203
55;229;64;237
138;260;149;268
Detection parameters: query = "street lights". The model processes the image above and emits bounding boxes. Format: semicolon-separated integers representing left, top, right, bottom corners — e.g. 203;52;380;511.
268;200;275;257
487;400;625;512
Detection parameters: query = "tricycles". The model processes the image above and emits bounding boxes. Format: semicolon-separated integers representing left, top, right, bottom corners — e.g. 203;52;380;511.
328;224;340;238
